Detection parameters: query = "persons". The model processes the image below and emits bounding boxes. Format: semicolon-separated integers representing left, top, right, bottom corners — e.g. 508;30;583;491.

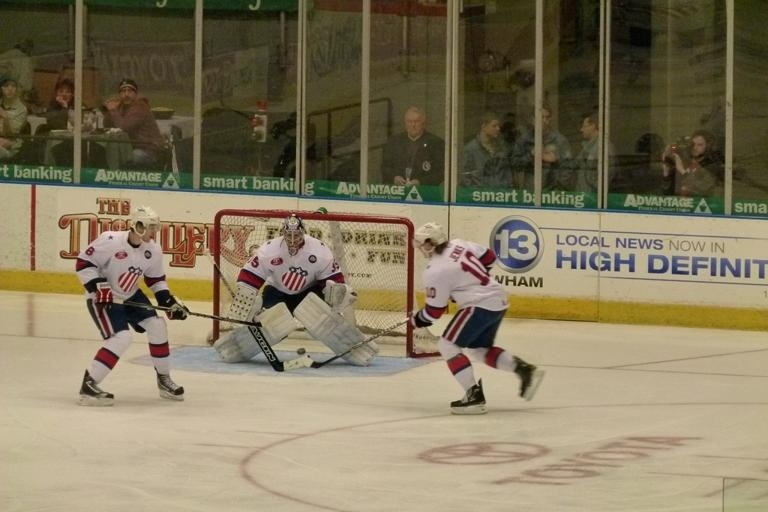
214;212;380;367
381;106;445;186
411;222;545;407
0;38;164;171
457;106;722;196
75;205;189;396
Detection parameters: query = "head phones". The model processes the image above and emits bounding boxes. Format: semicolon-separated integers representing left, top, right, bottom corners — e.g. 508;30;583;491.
701;130;715;152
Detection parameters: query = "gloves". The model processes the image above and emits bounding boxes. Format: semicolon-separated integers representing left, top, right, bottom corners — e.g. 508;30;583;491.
93;281;113;310
163;295;189;320
410;310;432;329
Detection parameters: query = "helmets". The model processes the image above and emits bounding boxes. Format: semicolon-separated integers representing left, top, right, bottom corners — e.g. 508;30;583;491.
284;216;304;255
131;206;160;236
414;223;448;254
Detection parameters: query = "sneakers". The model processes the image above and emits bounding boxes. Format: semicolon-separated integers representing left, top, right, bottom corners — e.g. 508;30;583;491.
451;388;486;406
157;375;184;395
80;381;114;398
514;362;536;396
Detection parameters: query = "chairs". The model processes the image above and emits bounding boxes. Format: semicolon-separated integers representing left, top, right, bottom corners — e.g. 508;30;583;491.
199;108;256;176
161;123;186;171
18;122;31;159
34;125;55;166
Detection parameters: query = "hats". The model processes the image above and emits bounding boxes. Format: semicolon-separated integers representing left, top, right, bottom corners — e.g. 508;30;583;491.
119;79;137;94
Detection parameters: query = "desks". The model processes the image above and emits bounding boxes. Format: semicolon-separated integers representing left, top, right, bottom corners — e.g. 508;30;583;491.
149;112;205;142
45;130;134;169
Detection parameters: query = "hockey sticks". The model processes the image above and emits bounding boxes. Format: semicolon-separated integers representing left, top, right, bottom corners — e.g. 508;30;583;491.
302;316;413;369
202;249;309;373
92;296;262;327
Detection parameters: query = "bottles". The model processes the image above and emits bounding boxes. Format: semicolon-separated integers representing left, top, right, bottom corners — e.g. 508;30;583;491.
67;103;104;135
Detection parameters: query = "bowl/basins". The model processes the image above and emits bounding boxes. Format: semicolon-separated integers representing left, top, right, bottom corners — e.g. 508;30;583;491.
151;108;174;120
51;128;68;137
